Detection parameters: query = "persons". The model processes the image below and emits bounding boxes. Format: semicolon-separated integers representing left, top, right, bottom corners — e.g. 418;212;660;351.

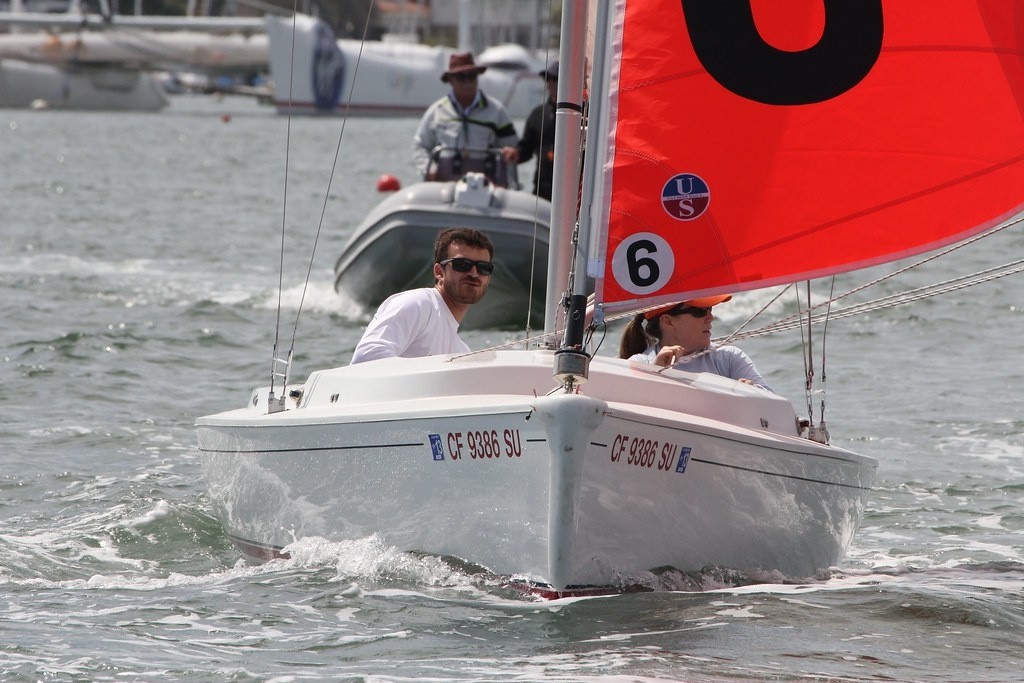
350;228;496;366
619;294;773;395
414;52;587;204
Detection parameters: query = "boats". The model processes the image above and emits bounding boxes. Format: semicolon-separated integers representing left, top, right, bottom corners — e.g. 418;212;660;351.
0;0;553;116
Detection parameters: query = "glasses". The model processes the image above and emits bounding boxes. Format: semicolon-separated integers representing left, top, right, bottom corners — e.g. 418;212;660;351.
438;257;494;275
450;72;477;80
670;305;712;318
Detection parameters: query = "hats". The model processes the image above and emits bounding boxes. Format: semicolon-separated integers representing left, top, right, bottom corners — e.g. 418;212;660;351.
440;53;487;83
539;62;559;78
644;294;732;319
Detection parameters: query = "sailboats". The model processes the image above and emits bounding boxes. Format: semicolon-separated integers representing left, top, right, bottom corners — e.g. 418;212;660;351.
193;0;1024;586
